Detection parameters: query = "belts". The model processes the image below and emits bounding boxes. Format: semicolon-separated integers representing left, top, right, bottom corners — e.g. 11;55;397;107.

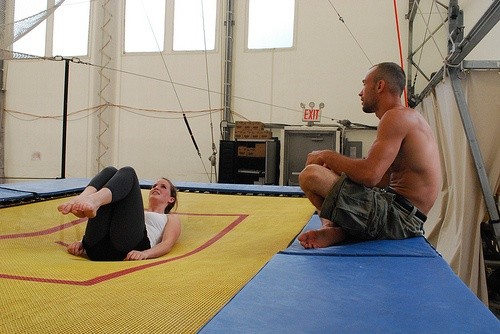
393;195;428;222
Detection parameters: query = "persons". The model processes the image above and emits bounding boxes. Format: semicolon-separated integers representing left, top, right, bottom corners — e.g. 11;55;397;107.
57;166;181;261
298;62;442;249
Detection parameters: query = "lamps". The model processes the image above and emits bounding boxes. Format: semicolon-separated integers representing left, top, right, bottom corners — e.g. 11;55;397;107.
300;102;325;126
